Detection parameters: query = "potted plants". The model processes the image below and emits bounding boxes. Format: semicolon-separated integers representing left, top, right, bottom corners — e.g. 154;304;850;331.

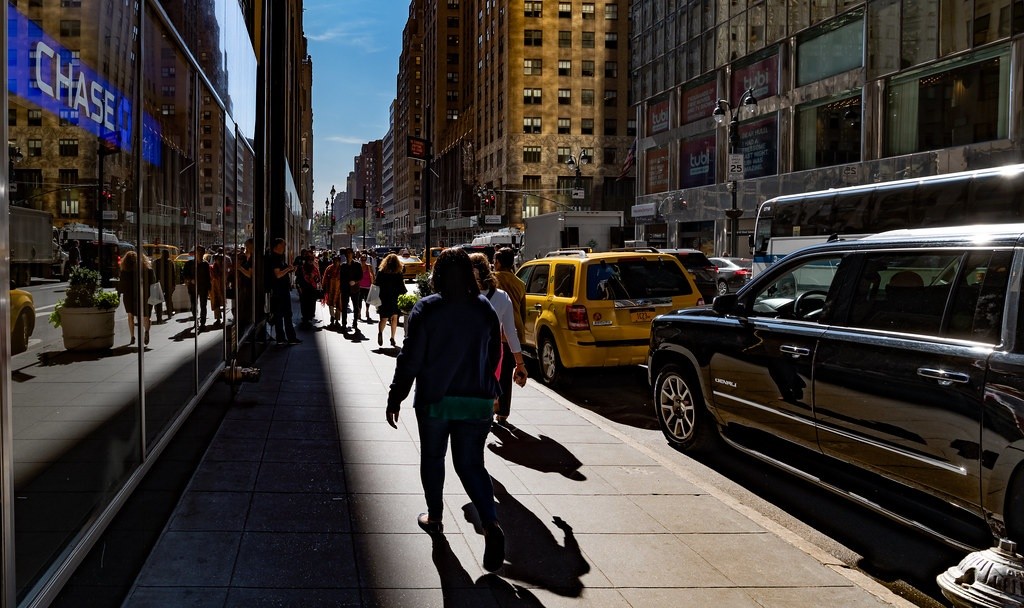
47;267;121;351
171;260;194;311
397;294;422;335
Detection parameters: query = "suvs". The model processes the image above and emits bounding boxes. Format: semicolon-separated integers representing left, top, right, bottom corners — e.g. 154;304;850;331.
513;249;706;387
610;248;720;306
645;234;1024;557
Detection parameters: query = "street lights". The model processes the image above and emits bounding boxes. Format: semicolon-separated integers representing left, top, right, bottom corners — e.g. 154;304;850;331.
329;184;337;250
477;184;488;234
8;142;24;206
712;87;760;259
115;176;128;242
324;198;330;251
566;148;590;212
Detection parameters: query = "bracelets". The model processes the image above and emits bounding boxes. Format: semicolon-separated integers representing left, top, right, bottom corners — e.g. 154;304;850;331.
516;363;526;366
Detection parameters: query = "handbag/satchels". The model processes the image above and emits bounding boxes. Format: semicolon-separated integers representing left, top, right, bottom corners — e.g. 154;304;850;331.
365;284;382;307
147;281;165;305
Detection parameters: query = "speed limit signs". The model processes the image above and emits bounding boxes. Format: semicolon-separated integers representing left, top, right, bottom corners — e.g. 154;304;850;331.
727;153;744;174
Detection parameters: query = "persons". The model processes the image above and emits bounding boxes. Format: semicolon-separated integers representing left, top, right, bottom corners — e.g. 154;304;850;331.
68;240;81;267
116;251;156;352
385;243;528;540
293;245;404;347
180;237;254;331
152;249;176;325
268;237;303;345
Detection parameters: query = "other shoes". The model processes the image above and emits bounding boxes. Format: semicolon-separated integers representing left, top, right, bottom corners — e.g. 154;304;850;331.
303;318;318;326
418;513;444;535
390;338;396;348
277;338;287;345
483;522;505;572
287;338;304;345
378;333;383;346
493;401;509;425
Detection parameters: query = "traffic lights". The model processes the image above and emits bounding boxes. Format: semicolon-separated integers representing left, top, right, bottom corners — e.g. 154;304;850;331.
182;209;187;218
103;189;116;205
490;194;496;209
485;196;489;207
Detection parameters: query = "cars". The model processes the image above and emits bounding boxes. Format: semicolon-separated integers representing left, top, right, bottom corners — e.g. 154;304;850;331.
59;220;233;284
372;232;524;281
8;280;35;353
707;255;752;296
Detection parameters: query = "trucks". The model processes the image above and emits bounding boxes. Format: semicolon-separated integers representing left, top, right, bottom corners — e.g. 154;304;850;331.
509;211;624;273
7;205;71;290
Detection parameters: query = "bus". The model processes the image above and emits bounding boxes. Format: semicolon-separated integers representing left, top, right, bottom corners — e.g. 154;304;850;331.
749;165;1023;300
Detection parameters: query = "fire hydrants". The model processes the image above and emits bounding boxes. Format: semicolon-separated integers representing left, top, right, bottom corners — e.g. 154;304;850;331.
935;538;1024;607
